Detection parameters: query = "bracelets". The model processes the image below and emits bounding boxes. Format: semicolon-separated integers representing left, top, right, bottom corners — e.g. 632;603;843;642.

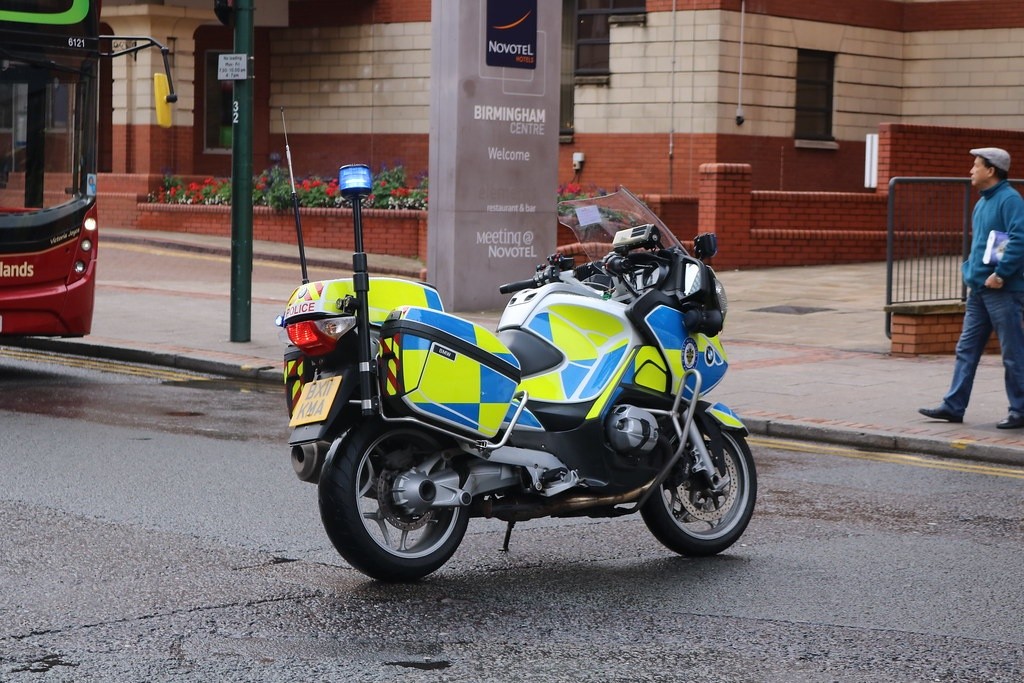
993;272;1003;283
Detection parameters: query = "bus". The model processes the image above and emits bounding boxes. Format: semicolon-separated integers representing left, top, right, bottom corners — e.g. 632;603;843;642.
0;0;181;342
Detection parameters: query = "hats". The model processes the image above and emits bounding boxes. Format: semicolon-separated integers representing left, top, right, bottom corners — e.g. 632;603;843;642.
969;148;1011;172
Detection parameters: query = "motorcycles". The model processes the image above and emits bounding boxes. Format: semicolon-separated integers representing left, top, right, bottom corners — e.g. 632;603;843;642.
274;161;759;579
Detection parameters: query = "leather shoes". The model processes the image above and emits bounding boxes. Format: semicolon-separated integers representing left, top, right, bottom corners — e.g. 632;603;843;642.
919;406;963;423
996;415;1024;429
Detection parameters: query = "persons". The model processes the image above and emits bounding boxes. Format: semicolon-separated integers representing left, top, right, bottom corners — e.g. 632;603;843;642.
918;148;1024;429
